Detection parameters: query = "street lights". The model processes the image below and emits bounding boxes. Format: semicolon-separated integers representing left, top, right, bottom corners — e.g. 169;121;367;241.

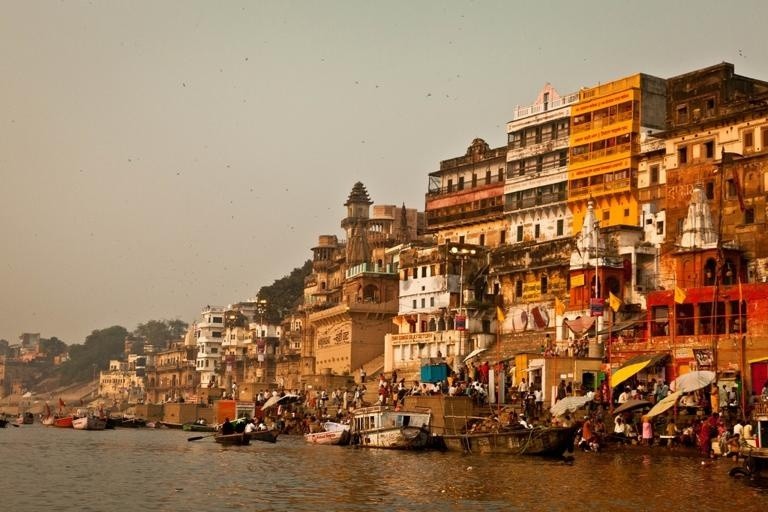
224;310;238;356
450;247;476;330
257;301;267;369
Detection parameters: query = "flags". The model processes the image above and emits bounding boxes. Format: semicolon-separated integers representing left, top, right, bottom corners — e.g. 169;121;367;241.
673;284;687;304
555;297;565;317
608;290;624;313
497;306;504;322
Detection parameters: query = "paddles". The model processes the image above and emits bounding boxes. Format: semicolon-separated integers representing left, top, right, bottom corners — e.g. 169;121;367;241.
6;422;19;428
187;432;221;442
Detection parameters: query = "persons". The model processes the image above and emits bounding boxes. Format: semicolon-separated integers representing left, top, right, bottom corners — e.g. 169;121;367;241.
207;366;767;463
535;332;589;362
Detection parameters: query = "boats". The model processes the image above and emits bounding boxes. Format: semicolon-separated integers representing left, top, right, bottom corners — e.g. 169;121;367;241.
42;405;161;430
0;418;8;428
214;428;280;446
303;405;578;456
16;414;33;424
